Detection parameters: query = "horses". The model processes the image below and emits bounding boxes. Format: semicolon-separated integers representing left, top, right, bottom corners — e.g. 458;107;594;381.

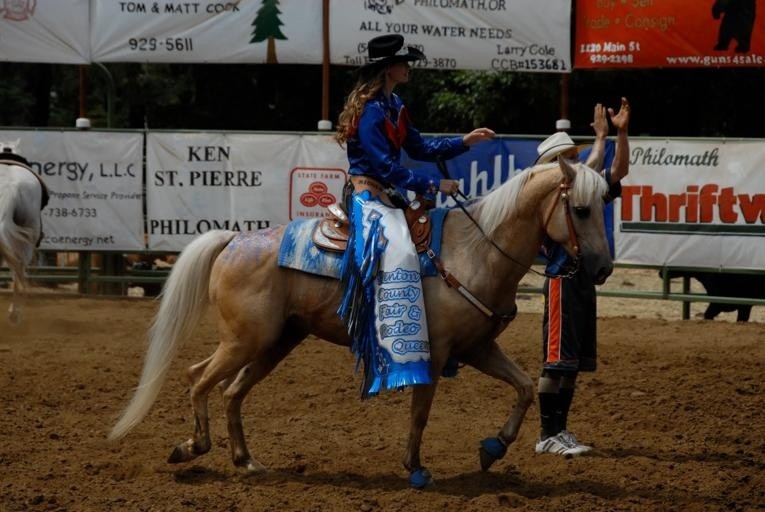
0;156;43;328
103;153;614;493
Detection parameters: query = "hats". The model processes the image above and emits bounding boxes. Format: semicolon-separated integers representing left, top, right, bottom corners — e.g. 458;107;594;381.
534;131;594;165
355;34;426;75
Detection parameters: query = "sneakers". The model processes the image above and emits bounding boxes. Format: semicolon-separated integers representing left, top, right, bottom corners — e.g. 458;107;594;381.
535;431;592;457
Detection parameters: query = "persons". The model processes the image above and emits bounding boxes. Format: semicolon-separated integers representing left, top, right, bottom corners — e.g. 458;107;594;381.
335;34;495;378
535;97;630;456
